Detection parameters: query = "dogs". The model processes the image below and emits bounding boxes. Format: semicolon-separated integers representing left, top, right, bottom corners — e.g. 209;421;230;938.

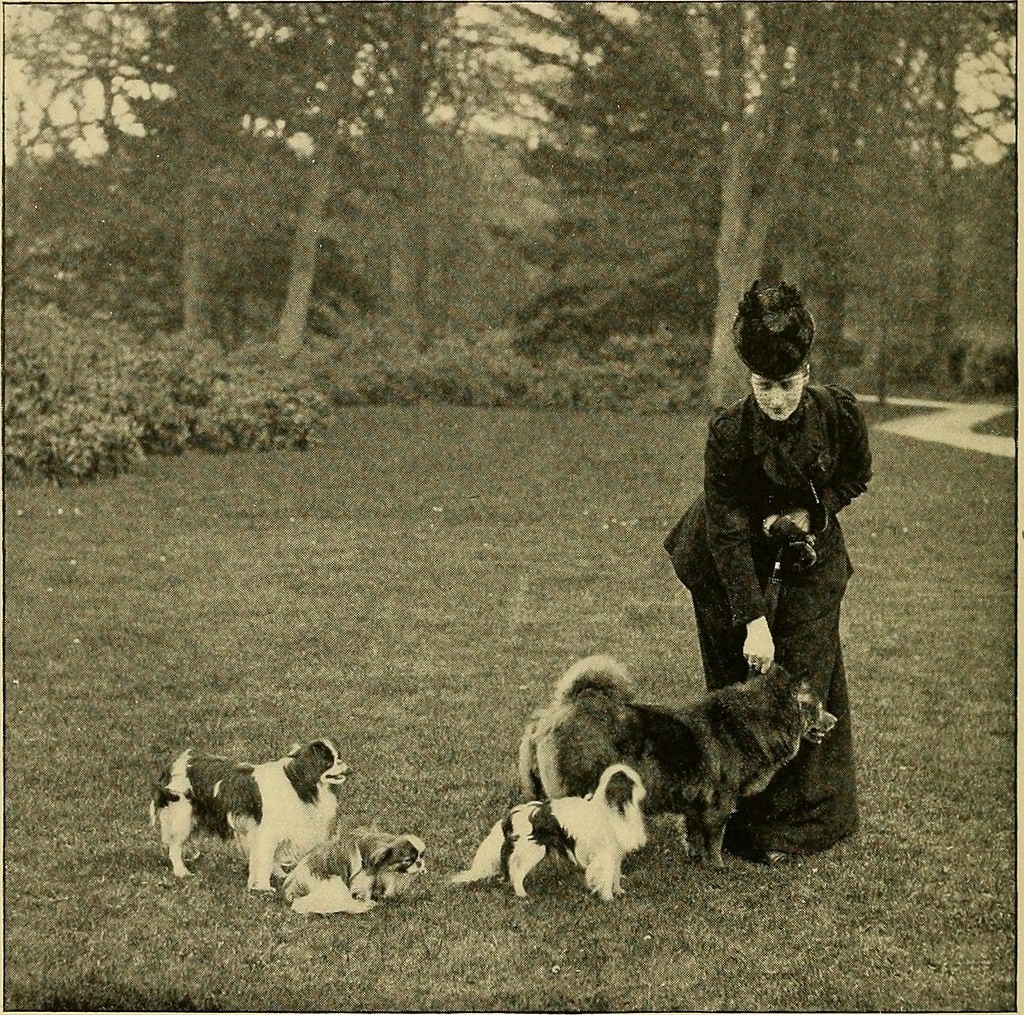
148;739;347;890
518;655;837;868
452;763;648;903
281;829;425;916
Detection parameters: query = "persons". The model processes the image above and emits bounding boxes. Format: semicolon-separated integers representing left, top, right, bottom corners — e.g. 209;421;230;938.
666;282;873;863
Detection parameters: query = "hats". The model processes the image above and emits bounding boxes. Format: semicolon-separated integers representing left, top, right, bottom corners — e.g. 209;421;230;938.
730;278;818;381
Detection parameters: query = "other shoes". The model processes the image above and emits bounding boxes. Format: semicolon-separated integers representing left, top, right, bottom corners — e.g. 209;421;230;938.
761;851;788;867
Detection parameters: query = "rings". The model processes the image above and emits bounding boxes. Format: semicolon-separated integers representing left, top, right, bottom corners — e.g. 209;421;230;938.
754;662;761;668
